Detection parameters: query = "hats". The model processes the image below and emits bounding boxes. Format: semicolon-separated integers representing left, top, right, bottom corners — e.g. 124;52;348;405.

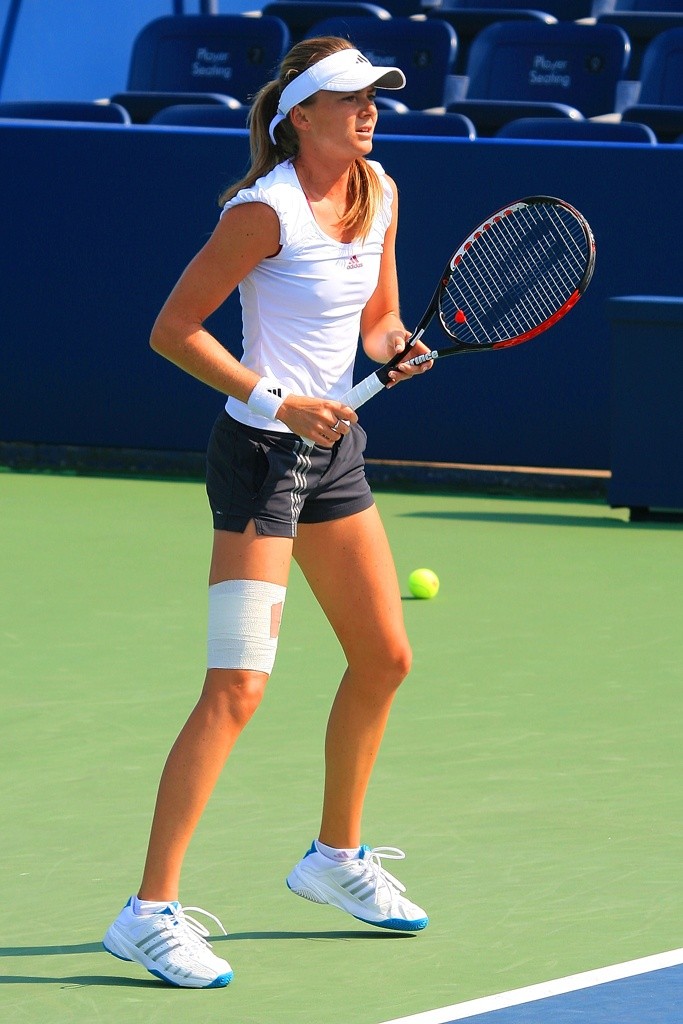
268;50;406;147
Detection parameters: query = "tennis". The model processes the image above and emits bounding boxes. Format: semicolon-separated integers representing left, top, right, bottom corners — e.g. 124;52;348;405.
408;568;440;598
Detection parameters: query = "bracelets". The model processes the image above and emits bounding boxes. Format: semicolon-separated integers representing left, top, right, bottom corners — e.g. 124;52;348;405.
247;377;292;421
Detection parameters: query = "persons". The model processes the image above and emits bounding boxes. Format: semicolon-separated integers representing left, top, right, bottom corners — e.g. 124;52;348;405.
103;36;434;988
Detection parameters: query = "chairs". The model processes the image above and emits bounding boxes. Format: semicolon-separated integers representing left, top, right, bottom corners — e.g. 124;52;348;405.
0;0;683;146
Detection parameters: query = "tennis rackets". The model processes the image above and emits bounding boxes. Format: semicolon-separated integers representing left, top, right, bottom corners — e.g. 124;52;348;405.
329;195;596;411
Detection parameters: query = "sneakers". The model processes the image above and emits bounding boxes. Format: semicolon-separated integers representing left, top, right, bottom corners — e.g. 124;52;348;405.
103;896;233;989
286;838;429;931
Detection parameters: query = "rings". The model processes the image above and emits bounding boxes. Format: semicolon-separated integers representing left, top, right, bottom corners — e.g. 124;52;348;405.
333;420;340;429
